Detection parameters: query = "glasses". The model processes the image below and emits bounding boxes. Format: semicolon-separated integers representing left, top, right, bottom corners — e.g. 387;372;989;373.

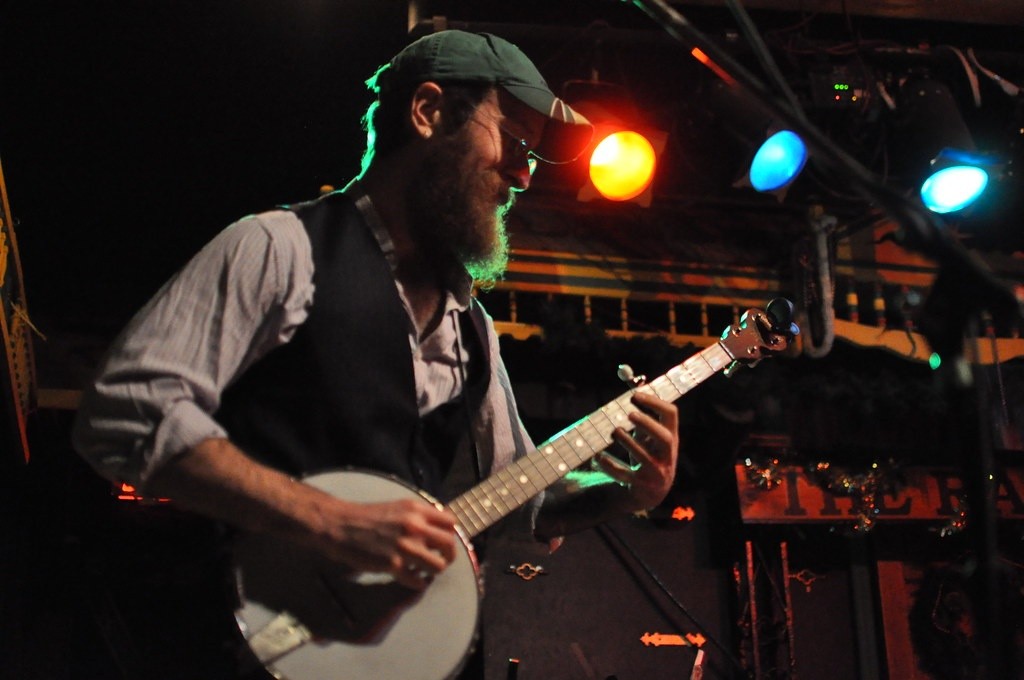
442;89;538;176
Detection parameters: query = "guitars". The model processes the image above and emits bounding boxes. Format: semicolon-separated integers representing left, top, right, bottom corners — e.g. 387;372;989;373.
221;293;801;680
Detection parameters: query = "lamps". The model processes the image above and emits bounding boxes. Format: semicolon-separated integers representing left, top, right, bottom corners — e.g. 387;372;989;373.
887;52;993;214
561;48;668;204
695;62;809;193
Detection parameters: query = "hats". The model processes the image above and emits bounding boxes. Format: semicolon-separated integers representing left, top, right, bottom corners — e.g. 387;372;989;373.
374;27;595;165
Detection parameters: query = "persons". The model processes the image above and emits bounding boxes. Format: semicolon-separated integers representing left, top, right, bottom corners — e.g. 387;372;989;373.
72;29;678;680
